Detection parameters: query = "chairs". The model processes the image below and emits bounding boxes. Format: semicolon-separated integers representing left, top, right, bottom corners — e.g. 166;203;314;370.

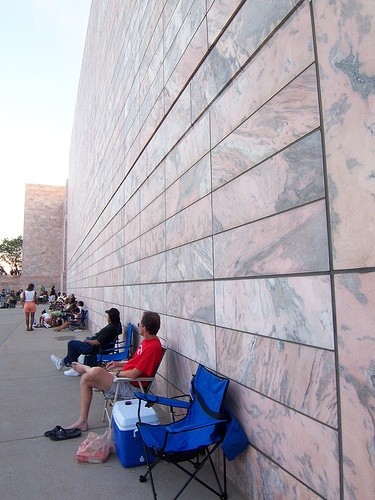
102;334;170;427
69;309;88;331
133;365;250;500
85;334;120;367
96;322;134;368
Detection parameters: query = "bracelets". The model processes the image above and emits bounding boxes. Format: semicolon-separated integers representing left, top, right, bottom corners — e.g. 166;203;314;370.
116;370;120;377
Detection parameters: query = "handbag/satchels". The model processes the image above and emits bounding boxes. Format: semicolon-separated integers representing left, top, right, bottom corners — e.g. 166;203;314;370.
77;428;112;463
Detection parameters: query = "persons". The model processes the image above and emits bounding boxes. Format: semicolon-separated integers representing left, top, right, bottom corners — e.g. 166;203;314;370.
21;283;37;331
0;288;23;307
63;310;163;433
38;285;86;332
50;307;123;376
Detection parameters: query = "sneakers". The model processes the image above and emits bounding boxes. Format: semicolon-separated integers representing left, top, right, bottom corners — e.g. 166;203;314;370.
51;354;63;370
64;367;80;377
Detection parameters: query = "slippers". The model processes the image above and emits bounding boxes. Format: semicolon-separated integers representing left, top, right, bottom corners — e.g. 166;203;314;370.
44;426;63;436
50;430;82;441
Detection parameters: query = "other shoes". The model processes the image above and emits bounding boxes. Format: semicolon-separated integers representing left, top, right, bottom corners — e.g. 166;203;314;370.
26;328;33;331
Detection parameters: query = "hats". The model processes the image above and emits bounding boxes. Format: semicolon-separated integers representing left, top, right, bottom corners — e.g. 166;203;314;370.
105;308;119;316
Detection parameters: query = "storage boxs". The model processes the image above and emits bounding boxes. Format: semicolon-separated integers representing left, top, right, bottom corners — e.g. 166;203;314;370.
110;397;160;469
